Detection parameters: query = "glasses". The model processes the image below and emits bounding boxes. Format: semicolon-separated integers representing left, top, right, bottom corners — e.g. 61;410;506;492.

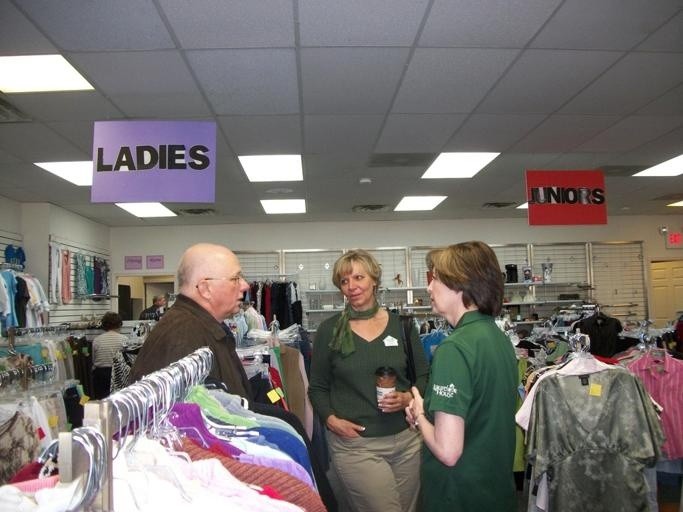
196;274;240;289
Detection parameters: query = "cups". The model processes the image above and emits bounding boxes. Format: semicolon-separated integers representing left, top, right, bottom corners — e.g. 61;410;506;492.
375;366;397;410
543;263;553;284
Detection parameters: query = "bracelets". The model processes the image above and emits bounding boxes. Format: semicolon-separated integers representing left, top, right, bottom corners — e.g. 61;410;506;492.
412;411;425;431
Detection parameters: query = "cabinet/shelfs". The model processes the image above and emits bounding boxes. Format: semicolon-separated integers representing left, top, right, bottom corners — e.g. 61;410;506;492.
305;281;583;332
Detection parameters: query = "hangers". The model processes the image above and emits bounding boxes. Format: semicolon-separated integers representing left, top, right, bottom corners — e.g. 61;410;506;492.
1;241;300;512
524;335;668;396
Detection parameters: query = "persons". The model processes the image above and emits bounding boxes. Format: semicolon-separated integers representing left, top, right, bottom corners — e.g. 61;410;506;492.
127;240;257;402
305;247;430;512
137;294;167;321
404;240;520;512
90;312;129;399
